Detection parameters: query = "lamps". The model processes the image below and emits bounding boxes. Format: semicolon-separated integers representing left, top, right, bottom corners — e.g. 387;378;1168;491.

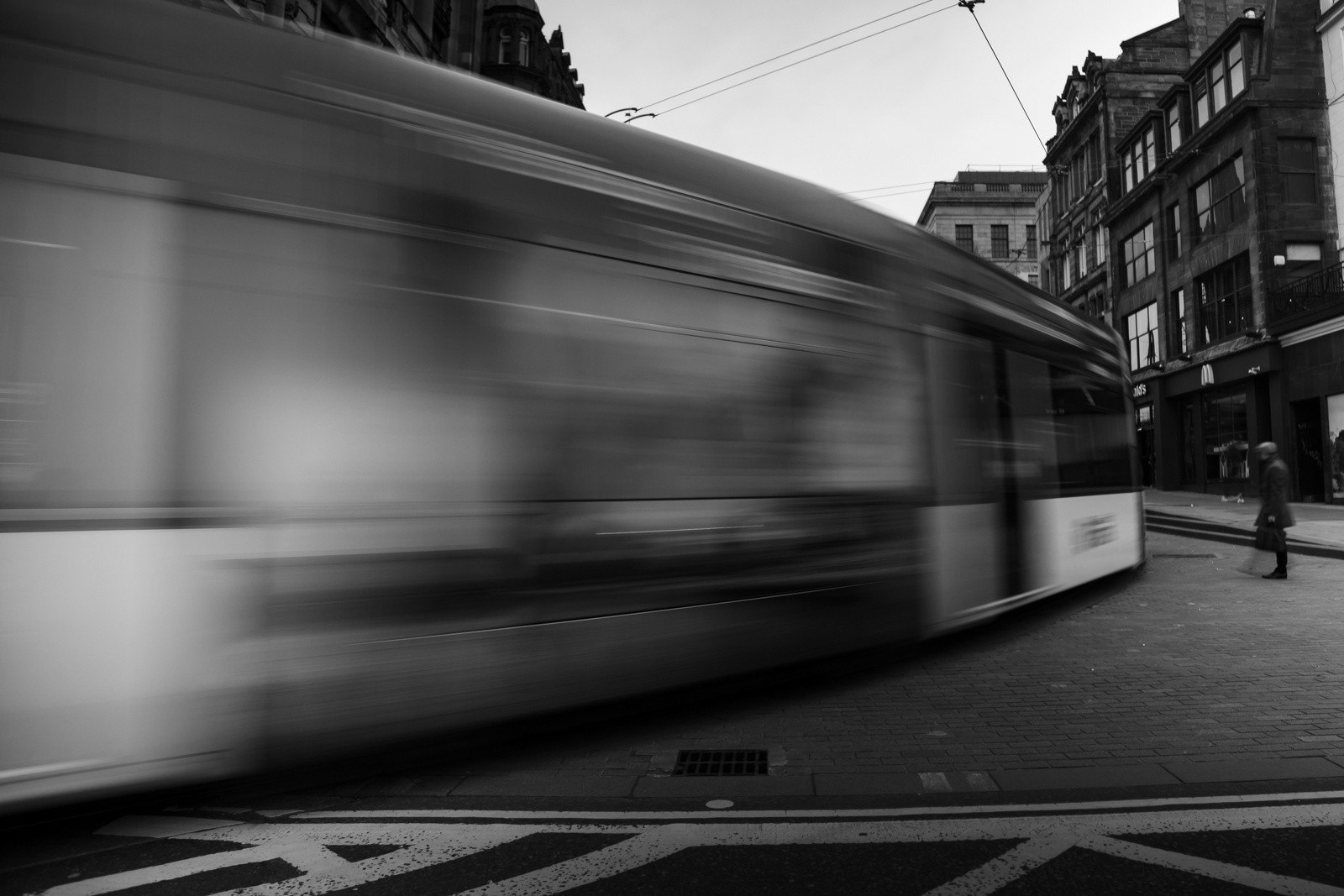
1245;327;1261;338
1178;353;1192;362
1151;363;1164;371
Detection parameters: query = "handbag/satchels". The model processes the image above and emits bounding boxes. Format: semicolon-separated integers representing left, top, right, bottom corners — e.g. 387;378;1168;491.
1254;528;1286;552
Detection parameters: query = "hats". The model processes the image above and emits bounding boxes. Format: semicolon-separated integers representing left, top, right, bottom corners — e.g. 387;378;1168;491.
1252;442;1277;453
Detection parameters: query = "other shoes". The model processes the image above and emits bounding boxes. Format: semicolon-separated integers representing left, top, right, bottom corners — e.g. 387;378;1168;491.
1261;568;1288;580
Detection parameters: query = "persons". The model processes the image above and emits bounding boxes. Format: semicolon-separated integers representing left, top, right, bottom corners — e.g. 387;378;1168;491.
1218;433;1250;503
1334;430;1344;493
1255;442;1296;579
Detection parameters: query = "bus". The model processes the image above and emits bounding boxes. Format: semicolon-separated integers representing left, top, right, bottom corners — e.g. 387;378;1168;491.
0;0;1147;809
